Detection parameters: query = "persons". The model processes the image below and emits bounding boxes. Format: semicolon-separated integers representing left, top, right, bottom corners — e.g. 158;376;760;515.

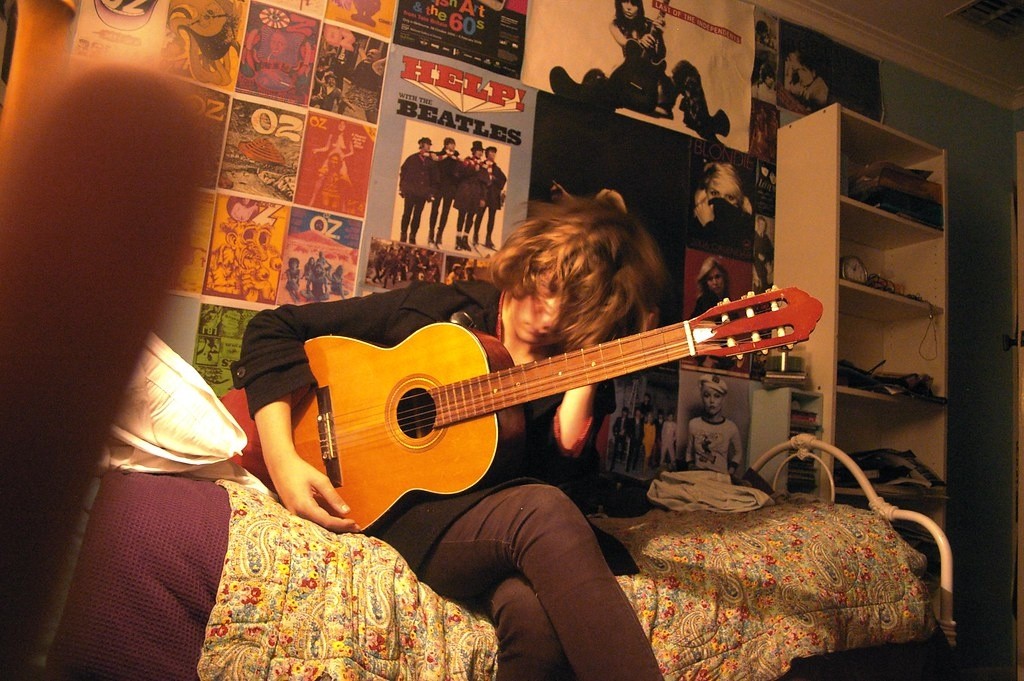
228;196;670;681
610;392;677;470
684;374;743;475
373;245;441;291
446;259;479;285
688;159;755;251
688;257;738;369
749;61;776;106
783;48;830;106
397;135;507;255
548;0;666;109
753;216;774;274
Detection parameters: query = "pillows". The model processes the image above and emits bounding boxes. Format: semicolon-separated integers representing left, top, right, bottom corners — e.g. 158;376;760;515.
109;332;248;466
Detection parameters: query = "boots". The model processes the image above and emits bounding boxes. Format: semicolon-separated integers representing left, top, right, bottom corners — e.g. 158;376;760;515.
461;236;472;251
456;236;463;250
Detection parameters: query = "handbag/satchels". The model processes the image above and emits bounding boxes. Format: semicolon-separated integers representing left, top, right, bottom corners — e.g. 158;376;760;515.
98;331;249;473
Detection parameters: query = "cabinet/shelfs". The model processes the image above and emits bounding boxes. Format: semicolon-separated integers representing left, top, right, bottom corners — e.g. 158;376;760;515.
771;103;947;618
749;388;823;497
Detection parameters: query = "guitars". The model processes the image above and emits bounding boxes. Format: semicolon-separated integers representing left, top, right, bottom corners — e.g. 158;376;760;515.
610;1;670;115
287;286;827;535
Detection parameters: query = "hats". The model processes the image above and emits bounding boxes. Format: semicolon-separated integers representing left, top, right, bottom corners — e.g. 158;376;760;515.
695;257;717;281
484;146;498;153
325;73;338;79
698;373;730;395
471;140;486;151
417;137;432;145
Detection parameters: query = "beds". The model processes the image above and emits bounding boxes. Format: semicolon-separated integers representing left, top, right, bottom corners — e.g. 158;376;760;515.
28;432;955;681
